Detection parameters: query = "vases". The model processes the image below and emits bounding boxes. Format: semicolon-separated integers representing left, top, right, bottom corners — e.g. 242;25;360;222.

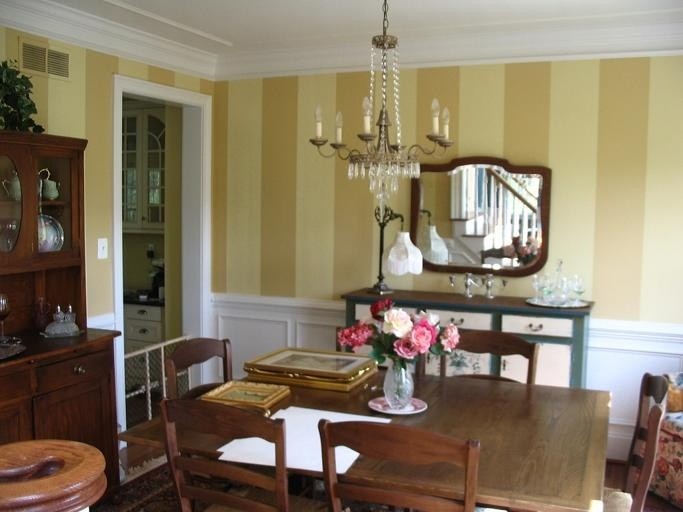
382;354;416;409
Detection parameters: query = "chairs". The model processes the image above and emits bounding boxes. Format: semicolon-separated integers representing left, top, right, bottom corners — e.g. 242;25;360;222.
162;336;316;512
415;326;541;387
158;398;328;512
633;373;682;509
603;370;670;512
317;418;480;511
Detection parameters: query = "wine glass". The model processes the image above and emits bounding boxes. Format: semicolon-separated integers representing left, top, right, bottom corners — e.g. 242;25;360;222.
530;271;586;307
0;219;19;251
0;294;10;344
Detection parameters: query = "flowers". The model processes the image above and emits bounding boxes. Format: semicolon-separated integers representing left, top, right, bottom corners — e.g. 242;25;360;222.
335;297;461;399
511;236;540;263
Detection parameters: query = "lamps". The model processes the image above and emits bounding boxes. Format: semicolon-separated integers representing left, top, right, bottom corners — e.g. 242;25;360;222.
365;204;424;295
309;1;455;222
418;208;449;266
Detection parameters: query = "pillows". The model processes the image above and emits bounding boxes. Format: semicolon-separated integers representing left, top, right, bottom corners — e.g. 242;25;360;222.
665;383;682;412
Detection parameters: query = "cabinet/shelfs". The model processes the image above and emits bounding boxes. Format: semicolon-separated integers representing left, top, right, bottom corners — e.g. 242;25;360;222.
0;130;121;506
123;300;164;393
121;107;181;234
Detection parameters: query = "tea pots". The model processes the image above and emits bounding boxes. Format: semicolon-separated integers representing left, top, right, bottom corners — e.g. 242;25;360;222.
2;168;22;201
38;167;61;201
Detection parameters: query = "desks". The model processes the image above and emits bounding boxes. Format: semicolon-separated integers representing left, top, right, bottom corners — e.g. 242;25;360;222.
340;287;596;389
114;369;613;512
0;439;108;512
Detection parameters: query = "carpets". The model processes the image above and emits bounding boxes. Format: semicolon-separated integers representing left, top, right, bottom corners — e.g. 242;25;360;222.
116;450;420;512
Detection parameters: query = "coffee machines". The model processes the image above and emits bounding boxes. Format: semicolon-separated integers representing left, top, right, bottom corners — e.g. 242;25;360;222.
149;260;165;300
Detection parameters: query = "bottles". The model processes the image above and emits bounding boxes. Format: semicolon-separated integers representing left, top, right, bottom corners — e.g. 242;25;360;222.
42;303;68;335
64;303;81;335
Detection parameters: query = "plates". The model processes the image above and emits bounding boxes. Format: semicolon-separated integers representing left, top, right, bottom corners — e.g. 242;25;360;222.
368;396;429;416
525;296;589;307
38;214;65;252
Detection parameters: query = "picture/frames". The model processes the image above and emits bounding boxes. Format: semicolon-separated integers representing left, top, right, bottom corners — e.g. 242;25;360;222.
198;379;291;412
246;367;377;393
243;347;377;380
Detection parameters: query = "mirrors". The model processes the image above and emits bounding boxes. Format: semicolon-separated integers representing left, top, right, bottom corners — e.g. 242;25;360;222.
408;154;552;278
